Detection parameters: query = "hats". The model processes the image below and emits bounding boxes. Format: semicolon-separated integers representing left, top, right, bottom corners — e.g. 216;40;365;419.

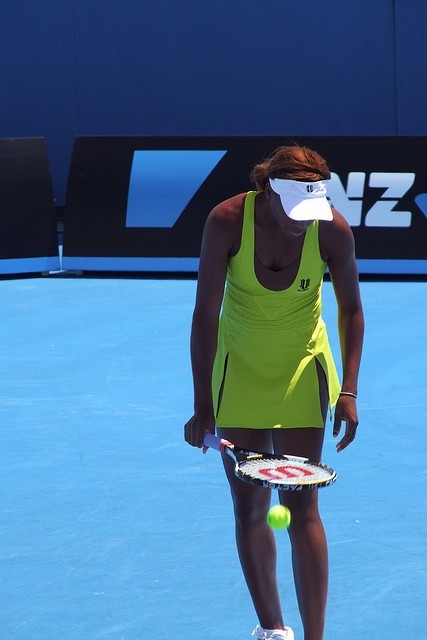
268;176;334;222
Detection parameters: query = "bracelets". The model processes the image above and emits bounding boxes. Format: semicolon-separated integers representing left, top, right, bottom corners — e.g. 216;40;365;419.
338;392;358;398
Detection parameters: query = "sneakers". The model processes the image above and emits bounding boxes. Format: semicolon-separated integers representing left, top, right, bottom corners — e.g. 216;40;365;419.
252;625;295;640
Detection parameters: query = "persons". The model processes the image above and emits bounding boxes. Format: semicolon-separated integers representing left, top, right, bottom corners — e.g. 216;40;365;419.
184;146;365;640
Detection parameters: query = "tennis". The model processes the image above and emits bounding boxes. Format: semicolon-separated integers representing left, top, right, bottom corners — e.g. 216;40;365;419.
266;504;292;530
